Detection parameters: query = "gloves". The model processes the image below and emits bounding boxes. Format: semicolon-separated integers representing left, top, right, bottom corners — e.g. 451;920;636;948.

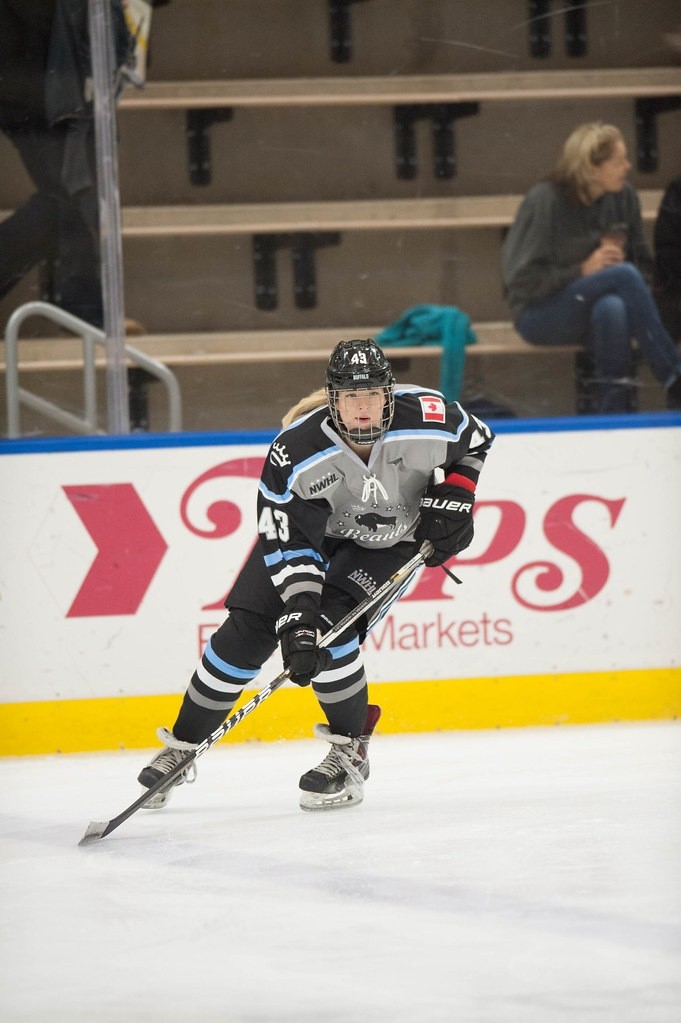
275;609;333;687
413;481;475;567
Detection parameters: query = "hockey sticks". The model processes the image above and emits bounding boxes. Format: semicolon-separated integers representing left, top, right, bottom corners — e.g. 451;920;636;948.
78;540;435;848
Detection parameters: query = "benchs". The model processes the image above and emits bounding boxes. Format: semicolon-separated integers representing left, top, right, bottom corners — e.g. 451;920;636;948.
86;66;681;189
0;318;638;434
0;189;669;314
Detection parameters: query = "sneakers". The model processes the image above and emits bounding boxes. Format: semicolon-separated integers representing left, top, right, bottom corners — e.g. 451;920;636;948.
299;705;381;812
137;728;197;809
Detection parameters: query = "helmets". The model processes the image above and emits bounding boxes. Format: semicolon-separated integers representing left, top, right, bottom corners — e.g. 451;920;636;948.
324;338;395;445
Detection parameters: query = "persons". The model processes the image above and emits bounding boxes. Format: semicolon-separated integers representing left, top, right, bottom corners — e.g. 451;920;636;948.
500;119;681;414
0;0;169;336
138;339;496;810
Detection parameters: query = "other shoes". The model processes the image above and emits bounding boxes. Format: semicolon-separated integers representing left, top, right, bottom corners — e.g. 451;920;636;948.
103;317;145;336
589;376;639;415
